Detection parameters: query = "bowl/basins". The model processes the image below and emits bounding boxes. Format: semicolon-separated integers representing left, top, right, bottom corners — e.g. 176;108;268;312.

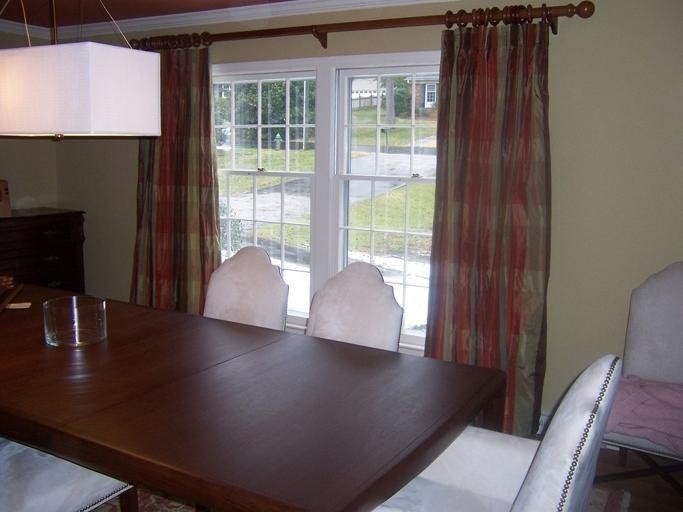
40;294;110;347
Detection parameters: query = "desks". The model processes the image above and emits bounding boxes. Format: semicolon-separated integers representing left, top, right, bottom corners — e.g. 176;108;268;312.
0;282;508;512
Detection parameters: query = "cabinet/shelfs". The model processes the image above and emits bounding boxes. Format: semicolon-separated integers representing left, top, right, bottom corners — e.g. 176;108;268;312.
0;206;88;295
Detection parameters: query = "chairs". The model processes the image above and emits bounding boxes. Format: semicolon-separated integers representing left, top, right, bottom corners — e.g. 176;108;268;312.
202;245;290;332
304;261;404;353
593;261;683;498
369;352;623;512
0;436;139;512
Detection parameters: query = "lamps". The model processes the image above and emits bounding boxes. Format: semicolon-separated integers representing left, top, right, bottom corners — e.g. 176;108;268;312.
0;0;162;138
